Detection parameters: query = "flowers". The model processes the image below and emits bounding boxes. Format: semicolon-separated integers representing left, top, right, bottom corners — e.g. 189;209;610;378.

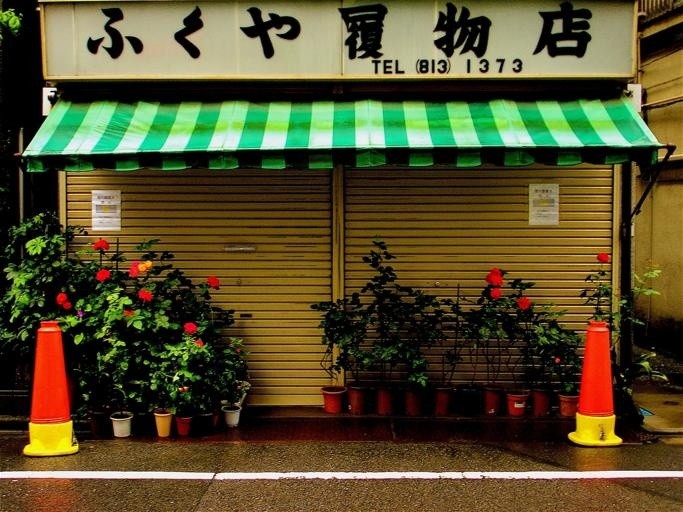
1;213;251;412
309;242;669;393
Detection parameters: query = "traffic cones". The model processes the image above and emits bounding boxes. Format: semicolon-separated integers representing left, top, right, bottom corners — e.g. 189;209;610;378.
566;320;624;447
20;317;84;457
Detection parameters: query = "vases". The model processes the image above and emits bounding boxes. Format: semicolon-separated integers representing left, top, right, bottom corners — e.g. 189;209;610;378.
109;412;134;437
211;415;220;427
320;385;580;416
153;408;173;438
221;405;242;428
175;416;192;437
